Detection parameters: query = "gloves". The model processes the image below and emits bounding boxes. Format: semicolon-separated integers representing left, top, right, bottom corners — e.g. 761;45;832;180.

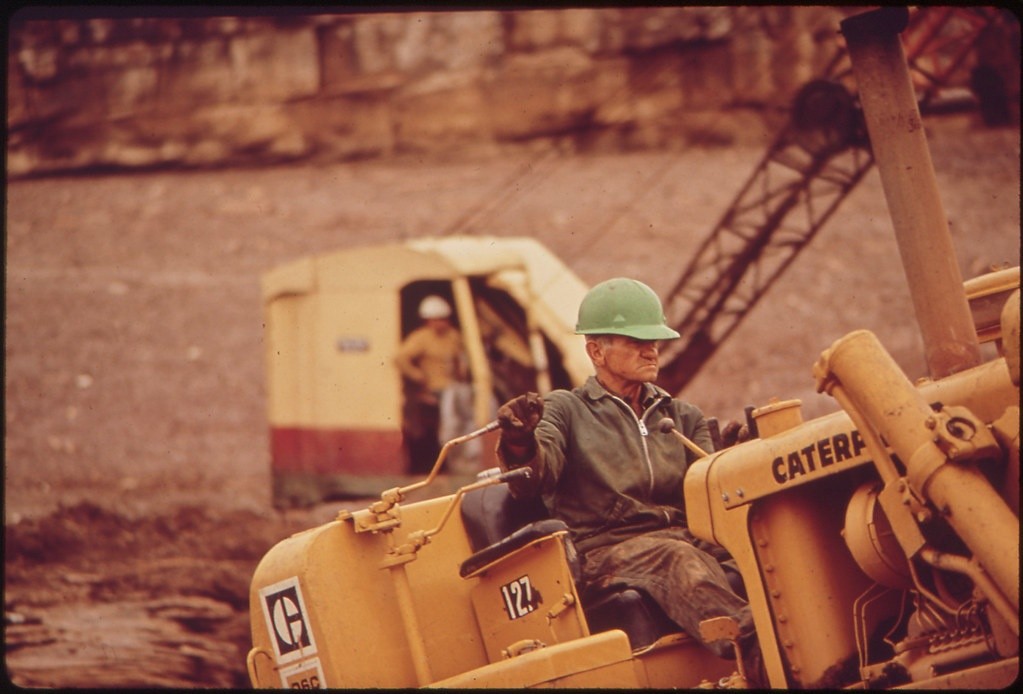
722;421;750;449
497;395;544;447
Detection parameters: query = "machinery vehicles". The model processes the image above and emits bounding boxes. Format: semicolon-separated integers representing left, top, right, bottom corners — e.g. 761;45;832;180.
247;0;1023;691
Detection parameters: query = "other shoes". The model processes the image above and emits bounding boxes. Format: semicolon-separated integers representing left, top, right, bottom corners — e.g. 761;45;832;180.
745;634;771;688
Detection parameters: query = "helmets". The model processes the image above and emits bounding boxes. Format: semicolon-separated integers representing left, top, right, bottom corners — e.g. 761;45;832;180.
574;278;680;339
417;295;451;319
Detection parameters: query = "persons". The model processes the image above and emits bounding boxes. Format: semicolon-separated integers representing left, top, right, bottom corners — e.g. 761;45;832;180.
495;277;754;660
394;294;470;445
